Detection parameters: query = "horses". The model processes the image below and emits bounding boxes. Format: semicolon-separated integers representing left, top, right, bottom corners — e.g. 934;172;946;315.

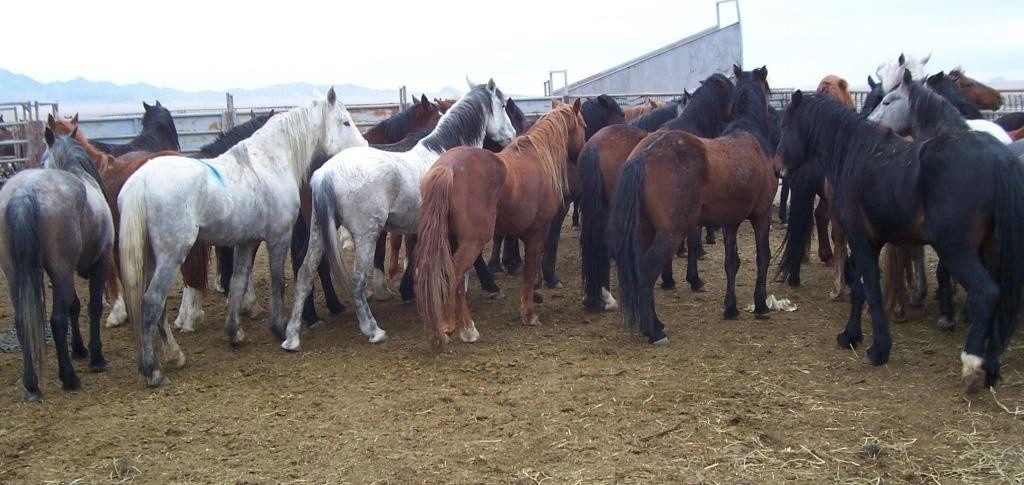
2;51;1024;205
117;86;369;389
2;126;114;401
407;97;587;353
576;73;734;311
280;77;516;351
399;94;626;300
606;64;777;346
291;94;445;330
772;90;1024;399
815;75;922;323
45;111;211;334
